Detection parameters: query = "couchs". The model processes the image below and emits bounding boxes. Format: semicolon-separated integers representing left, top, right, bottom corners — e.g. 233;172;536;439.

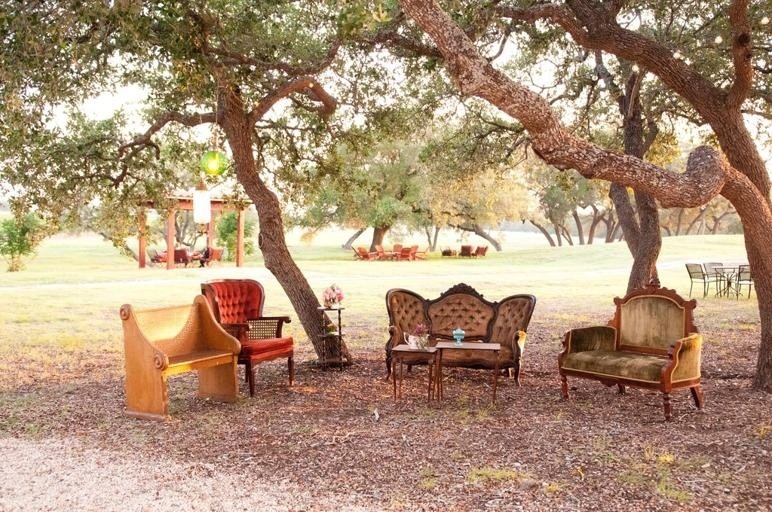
385;282;536;386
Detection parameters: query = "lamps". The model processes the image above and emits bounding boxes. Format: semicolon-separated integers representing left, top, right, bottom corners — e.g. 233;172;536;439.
192;102;212;225
200;69;226;177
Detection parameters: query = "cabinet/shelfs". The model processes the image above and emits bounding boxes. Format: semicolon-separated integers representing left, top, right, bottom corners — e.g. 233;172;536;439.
317;306;348;373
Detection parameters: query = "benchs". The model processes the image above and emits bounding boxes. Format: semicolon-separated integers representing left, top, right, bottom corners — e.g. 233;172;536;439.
120;295;242;421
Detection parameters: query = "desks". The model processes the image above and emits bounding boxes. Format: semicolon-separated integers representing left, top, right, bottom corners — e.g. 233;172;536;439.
390;344;438;402
442;249;456;256
435;340;501;405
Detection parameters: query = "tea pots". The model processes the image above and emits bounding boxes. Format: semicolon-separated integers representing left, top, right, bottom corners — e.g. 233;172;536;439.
403;332;430;350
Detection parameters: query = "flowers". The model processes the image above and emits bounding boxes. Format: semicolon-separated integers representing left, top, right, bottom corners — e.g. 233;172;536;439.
322;285;343;306
415;323;426;336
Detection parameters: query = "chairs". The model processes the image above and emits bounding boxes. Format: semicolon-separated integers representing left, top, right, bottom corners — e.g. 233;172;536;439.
353;243;419;261
685;262;752;301
559;287;704;421
147;245;224;267
458;245;489;256
201;279;294;398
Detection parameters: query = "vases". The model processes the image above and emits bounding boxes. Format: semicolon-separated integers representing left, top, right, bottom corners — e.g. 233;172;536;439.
417;334;430;351
330;299;340;309
